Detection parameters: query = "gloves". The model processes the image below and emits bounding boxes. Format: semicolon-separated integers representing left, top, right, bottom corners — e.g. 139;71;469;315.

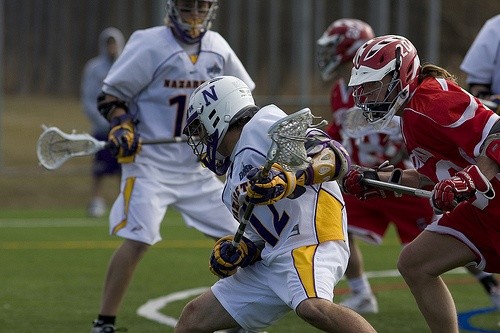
430;164;495;215
107;115;142;163
209;235;265;279
477;91;500;114
247;162;314;206
342;160;403;199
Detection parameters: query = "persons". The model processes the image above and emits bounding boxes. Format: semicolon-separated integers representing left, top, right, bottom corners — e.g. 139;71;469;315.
92;0;255;333
318;18;500;313
460;14;500;102
173;75;379;333
340;35;500;333
82;28;124;214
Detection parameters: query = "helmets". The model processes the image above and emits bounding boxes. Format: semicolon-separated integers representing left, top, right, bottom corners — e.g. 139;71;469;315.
166;0;218;44
183;76;260;176
317;18;376;82
348;34;421;120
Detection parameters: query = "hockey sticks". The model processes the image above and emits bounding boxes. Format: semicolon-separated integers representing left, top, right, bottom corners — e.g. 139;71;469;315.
36;126;202;170
230;107;312;253
341;97;498;142
342;164;432;200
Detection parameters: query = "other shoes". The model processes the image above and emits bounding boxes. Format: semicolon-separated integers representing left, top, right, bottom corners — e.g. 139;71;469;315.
91;319;116;333
89;195;105;217
339;293;378;314
